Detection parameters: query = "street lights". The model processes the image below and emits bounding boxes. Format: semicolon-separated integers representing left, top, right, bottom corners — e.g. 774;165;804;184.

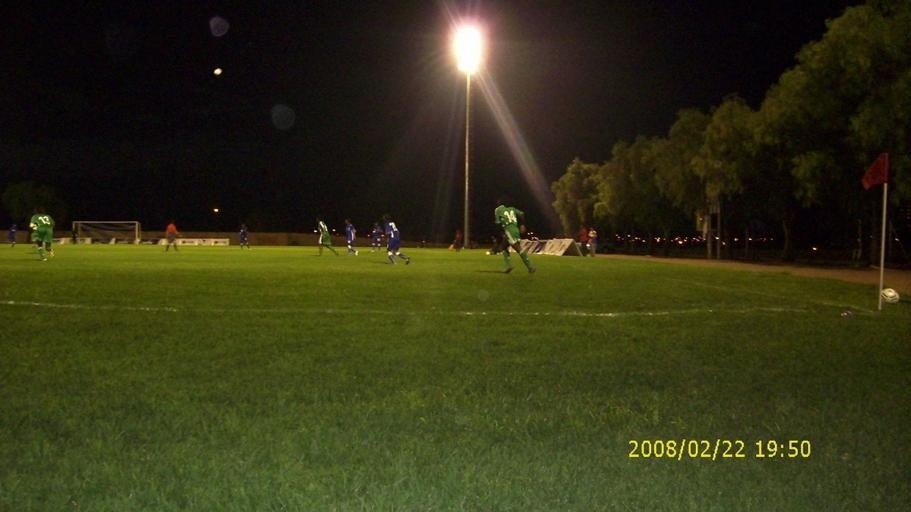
448;16;490;249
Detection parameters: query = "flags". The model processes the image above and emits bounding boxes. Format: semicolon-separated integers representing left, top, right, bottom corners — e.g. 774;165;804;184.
860;149;889;191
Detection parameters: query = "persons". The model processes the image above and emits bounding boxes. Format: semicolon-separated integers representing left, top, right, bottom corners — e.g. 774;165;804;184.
28;205;56;263
237;223;250;249
587;226;600;255
165;219;181;252
384;215;414;266
314;215;340;256
487;222;535;257
7;223;18;246
576;223;588;255
344;216;360;257
368;222;386;254
493;199;537;275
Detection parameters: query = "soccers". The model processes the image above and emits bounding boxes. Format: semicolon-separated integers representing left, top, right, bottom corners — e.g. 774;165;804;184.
486;251;490;255
882;288;898;303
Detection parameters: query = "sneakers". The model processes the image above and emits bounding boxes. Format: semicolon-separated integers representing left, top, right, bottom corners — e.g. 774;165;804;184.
504;264;515;273
385;261;397;265
528;267;537;274
404;255;411;265
48;251;55;258
40;256;48;262
355;250;359;257
